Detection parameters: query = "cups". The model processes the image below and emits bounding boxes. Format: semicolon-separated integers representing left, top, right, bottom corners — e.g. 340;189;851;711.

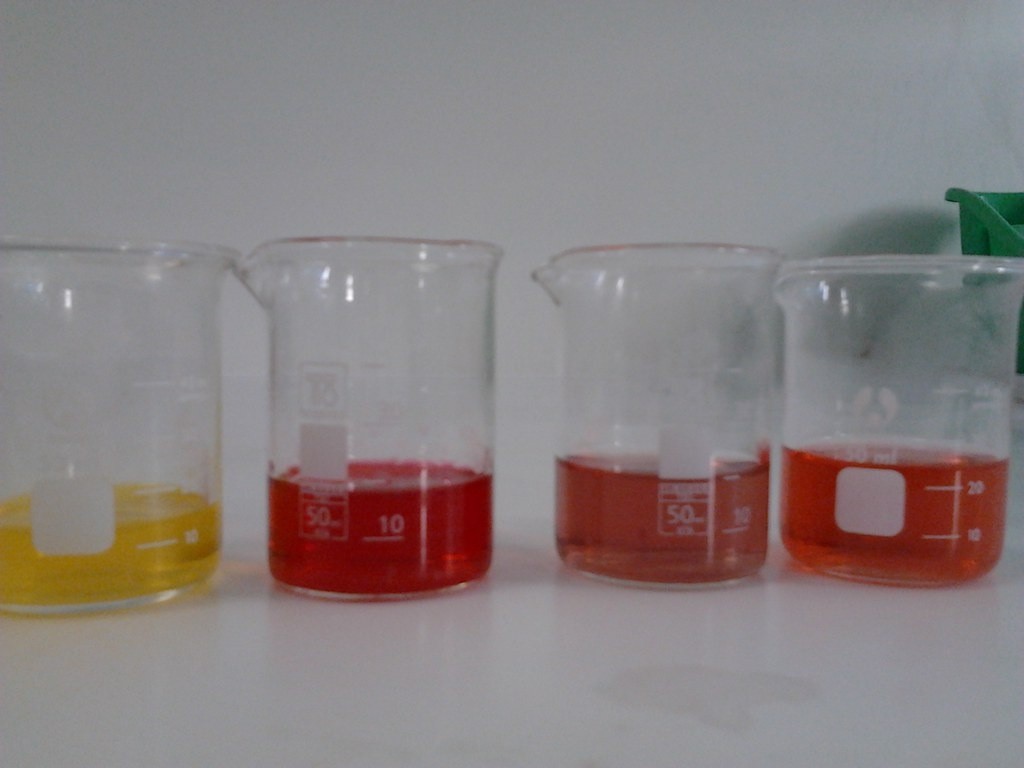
232;238;502;603
1;238;234;617
774;254;1024;586
531;241;775;591
